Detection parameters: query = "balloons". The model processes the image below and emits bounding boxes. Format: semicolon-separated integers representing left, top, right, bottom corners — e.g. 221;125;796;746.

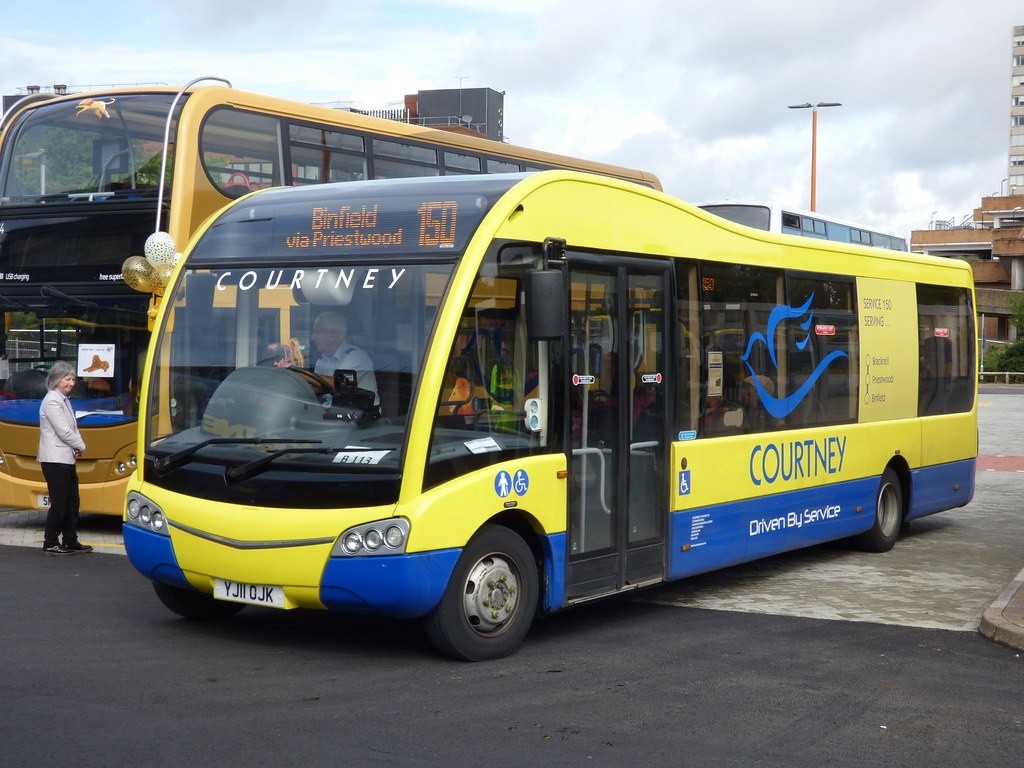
150;263;176;296
144;231;176;269
172;253;182;267
121;256;155;292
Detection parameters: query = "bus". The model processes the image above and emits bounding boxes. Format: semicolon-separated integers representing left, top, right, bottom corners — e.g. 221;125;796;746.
704;201;914;254
2;79;664;511
121;177;981;660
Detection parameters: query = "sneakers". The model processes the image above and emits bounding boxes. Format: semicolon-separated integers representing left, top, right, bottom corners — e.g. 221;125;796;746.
43;542;75;555
62;540;93;553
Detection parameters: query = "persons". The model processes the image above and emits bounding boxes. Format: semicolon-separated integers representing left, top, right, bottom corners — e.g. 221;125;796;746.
36;362;93;555
0;338;9;397
701;375;774;433
297;311;382;415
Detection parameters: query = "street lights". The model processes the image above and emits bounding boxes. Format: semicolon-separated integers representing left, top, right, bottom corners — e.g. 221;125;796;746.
788;100;843;213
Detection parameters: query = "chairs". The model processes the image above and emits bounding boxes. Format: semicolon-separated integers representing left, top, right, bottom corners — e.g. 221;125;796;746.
223;174;263;198
446;327;955;432
